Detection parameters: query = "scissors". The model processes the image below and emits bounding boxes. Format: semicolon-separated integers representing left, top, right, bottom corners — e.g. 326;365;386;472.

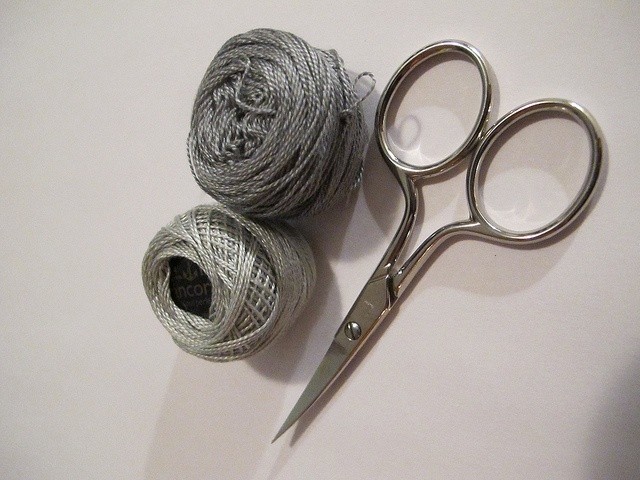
270;41;602;441
